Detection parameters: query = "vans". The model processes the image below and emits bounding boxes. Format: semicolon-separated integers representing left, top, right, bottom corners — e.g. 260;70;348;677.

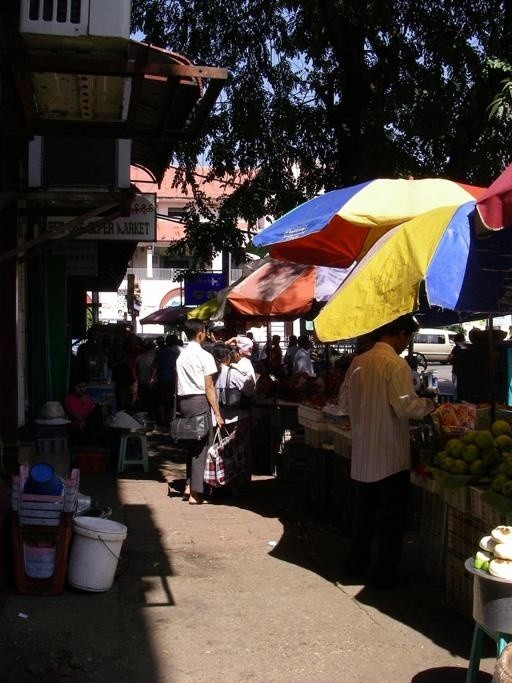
404;328;459;364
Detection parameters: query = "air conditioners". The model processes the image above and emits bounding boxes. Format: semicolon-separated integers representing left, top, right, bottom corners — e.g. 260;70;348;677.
19;1;135;123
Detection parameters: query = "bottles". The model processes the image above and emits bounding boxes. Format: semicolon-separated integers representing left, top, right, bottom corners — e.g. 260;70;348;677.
412;367;421;392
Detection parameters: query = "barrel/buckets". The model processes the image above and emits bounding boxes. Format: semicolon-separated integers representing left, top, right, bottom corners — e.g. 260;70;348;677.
69;515;128;593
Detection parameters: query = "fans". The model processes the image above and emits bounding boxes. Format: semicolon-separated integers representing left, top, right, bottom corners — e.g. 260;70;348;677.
405;350;427;376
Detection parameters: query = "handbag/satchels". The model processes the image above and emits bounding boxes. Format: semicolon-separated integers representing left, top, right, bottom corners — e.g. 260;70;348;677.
169;413;210;441
203;425;252;490
218;366;247;409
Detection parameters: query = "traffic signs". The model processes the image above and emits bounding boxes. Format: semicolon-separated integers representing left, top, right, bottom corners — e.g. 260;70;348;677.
183;268;227;307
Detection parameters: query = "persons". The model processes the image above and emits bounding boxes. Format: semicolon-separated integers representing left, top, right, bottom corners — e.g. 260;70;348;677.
75;317;511;509
335;312;440;595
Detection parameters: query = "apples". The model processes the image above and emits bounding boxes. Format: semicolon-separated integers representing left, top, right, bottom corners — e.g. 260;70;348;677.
432;420;512;499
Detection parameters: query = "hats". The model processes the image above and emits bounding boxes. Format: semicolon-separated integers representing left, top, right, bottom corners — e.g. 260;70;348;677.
235;334;254;356
206;320;229;332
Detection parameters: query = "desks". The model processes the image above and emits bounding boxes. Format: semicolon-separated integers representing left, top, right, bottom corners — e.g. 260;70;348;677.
34;422;74;477
87;380;117;409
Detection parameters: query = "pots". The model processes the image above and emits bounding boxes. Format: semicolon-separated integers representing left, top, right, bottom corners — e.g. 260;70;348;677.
463;555;512;639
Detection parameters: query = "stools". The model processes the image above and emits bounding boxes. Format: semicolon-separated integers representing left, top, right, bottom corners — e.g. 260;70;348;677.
4;459;79;598
462;620;512;680
117;430;150;472
